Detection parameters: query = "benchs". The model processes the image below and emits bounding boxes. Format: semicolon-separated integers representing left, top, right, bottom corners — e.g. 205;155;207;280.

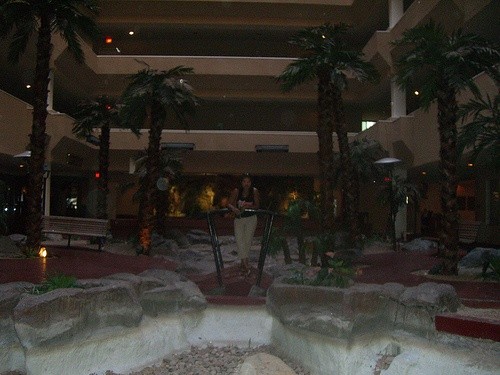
457;221;483;253
40;215;112;253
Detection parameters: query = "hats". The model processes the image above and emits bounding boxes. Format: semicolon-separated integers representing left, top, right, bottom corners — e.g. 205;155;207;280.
241;173;251;177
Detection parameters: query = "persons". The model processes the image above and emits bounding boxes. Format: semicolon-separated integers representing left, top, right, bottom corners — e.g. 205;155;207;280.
226;171;261;279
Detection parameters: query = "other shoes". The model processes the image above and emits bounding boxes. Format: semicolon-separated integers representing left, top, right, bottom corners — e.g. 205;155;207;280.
240;265;256;281
